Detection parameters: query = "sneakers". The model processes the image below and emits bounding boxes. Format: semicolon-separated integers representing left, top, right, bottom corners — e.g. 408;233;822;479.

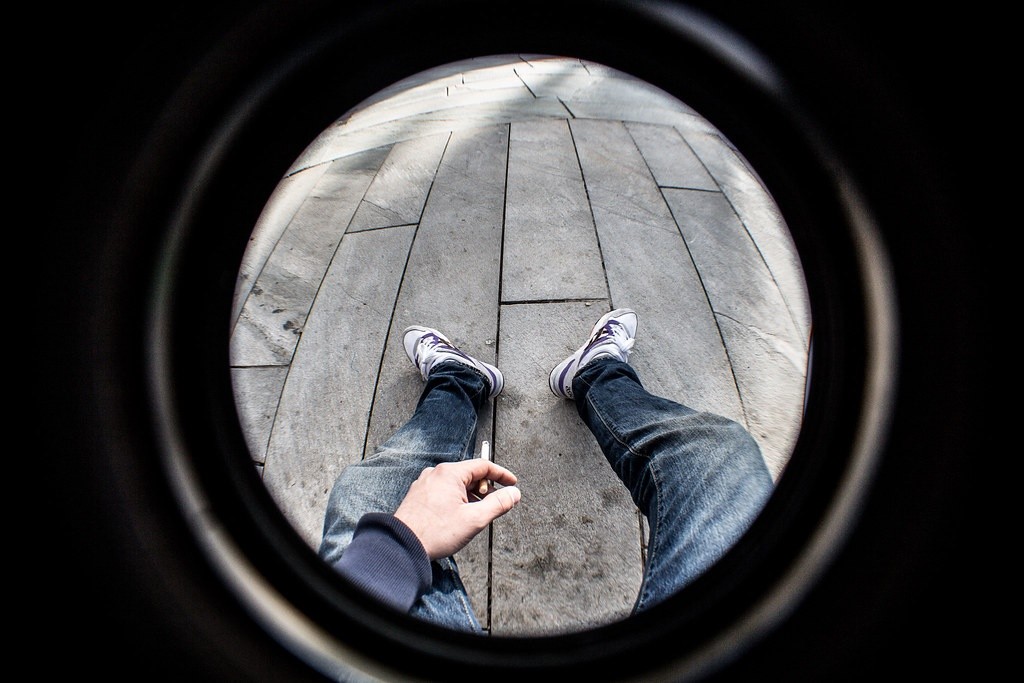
548;308;638;400
401;326;504;398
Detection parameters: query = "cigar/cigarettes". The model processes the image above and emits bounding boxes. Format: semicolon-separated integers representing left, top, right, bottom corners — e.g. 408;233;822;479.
479;441;490;494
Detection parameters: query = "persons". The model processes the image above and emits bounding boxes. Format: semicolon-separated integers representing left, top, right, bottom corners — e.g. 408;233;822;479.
316;308;774;636
